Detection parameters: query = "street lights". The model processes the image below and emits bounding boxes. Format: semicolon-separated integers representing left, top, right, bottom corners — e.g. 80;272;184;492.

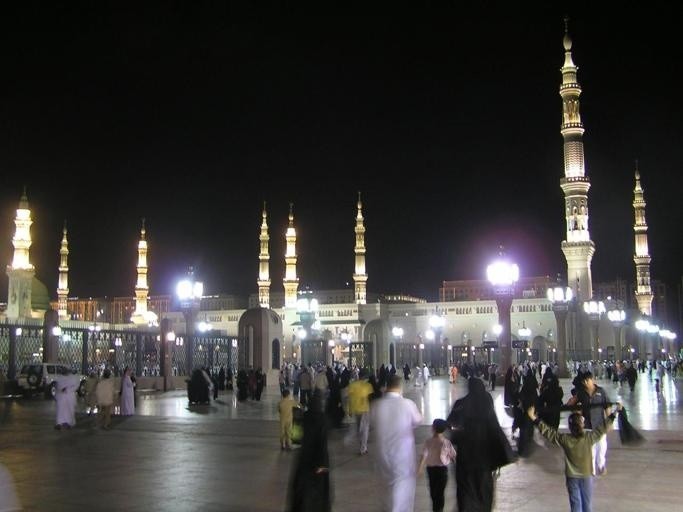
329;339;336;366
215;344;220;366
545;272;574;380
391;325;403;368
482;243;520;383
632;314;676;363
442;338;452;373
464;339;475;365
413;334;424;366
582;293;604;377
173;263;204;376
517;320;532;362
606;301;626;364
484;348;495;364
427;309;445;374
87;320;100;373
293;291;318;338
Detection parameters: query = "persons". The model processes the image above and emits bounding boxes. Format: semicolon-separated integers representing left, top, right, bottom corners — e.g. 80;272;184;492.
357;374;423;512
50;364;140;433
445;376;518;510
448;361;500;390
413;417;457;510
187;367;264;404
277;389;304;449
504;367;563;442
279;360;430;430
505;358;682;392
568;371;611;477
525;402;623;512
284;394;333;512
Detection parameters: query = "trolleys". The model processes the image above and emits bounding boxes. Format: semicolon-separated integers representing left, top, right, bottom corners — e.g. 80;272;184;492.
282;404;303;449
186;371;213;408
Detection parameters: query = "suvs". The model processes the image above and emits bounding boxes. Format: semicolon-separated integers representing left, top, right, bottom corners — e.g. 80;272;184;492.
16;364;90;400
